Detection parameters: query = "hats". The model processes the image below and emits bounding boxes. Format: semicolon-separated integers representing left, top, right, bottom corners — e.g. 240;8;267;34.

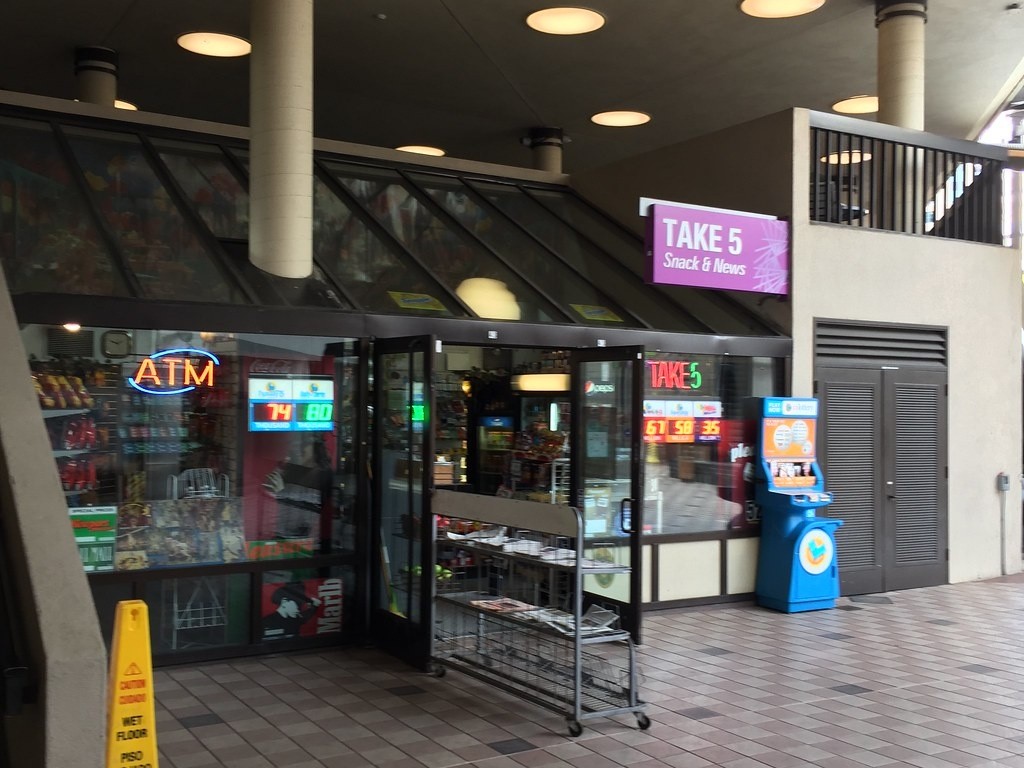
289;432;327;444
270;578;314;608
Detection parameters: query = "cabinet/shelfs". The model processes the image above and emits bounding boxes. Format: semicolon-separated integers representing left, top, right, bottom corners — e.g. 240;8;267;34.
389;354;650;736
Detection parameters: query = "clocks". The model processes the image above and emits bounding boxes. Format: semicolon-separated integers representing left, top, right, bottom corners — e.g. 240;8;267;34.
102;330;133;358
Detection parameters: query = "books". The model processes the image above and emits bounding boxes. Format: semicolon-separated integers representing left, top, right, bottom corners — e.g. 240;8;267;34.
448;528;627;569
472;597;616;636
